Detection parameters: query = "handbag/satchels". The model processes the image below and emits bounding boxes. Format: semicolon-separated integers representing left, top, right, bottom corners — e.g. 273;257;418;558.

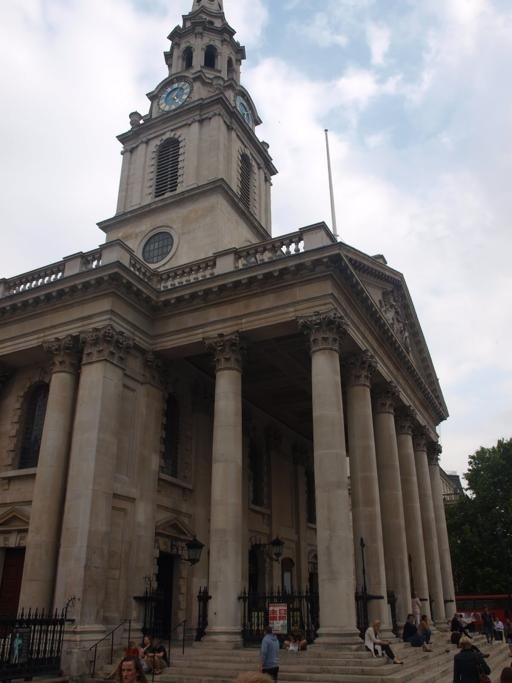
475;653;492;682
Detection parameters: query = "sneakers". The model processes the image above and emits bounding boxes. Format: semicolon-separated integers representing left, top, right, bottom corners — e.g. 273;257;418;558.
393;661;403;664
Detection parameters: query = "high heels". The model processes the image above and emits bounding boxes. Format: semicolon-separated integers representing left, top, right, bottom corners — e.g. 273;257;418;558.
423;645;432;652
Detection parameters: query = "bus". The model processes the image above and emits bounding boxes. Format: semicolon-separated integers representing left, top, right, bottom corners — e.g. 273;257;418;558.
456;594;511;633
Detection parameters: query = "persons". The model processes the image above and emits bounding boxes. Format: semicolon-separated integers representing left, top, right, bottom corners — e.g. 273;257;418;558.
452;612;472;639
141;636;170;674
235;670;276;683
480;605;493;643
456;614;470;627
499;666;511;683
281;622;307;651
452;636;491;682
449;620;460;633
363;618;404;664
450;626;463;647
12;632;23;664
118;655;149;683
416;614;433;644
258;625;280;683
402;613;434;651
126;640;140;658
136;635;153;674
492;615;504;630
500;613;512;658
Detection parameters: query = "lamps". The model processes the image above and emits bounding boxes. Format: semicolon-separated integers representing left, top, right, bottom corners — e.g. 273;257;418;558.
270;532;285;561
181;532;205;565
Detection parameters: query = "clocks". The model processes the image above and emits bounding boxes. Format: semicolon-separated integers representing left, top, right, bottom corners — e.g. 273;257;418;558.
156;75;193;111
234;91;254;131
